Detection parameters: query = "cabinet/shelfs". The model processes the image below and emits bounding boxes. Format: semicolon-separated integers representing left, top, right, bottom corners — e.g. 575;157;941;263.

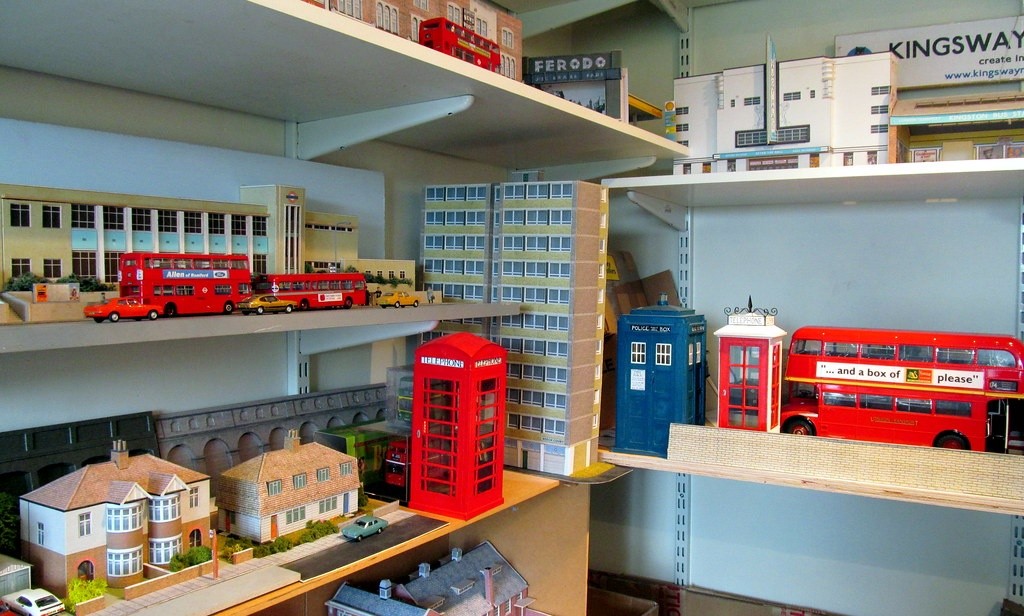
596;154;1024;521
0;0;694;616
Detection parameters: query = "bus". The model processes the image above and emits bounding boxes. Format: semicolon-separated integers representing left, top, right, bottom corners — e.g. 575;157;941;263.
384;438;446;488
419;17;502;71
781;327;1024;454
119;253;252;317
256;273;366;309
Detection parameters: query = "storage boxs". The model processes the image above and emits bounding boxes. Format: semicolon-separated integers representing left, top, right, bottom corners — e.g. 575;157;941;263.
587;587;659;616
604;249;650;335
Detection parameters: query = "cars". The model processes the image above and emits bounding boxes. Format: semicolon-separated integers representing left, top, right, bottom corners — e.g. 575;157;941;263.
377;291;421;307
342;516;388;541
237;295;297;315
0;588;65;616
84;298;164;322
